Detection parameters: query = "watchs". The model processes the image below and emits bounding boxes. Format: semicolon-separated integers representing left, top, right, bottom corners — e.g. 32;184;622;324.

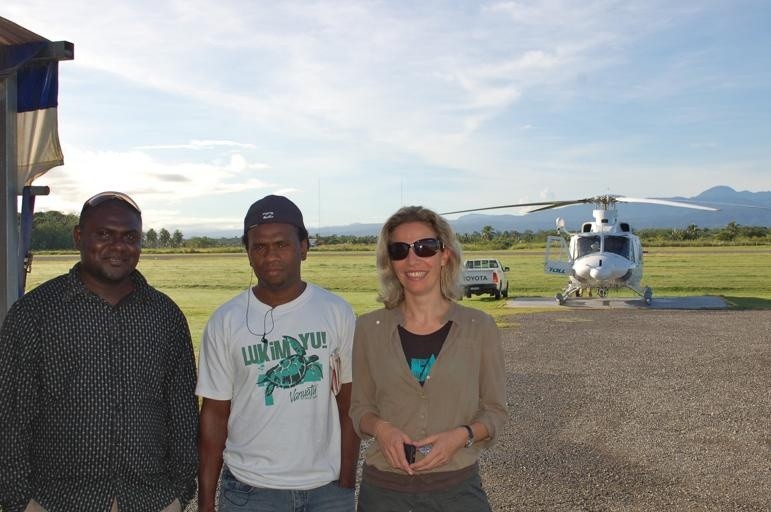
462;424;476;448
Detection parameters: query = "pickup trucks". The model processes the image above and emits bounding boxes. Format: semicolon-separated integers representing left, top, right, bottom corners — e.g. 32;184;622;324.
461;259;510;300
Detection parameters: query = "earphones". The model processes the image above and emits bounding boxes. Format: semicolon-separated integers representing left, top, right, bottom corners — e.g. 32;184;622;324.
259;335;268;342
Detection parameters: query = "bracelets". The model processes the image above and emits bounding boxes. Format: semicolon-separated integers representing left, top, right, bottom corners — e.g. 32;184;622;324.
373;420;391;438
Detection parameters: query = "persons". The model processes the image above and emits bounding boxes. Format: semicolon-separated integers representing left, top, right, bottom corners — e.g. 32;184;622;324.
345;208;510;512
0;189;202;512
196;194;359;512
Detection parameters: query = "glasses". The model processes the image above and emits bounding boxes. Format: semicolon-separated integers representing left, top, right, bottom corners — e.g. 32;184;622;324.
386;237;445;261
84;190;142;216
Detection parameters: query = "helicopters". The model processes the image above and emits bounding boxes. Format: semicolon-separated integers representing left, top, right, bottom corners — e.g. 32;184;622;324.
438;194;767;305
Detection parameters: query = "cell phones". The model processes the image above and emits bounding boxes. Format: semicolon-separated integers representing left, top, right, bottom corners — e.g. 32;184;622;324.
401;442;417;464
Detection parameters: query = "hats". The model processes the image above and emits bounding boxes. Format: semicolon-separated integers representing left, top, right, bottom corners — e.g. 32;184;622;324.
242;194;306;235
